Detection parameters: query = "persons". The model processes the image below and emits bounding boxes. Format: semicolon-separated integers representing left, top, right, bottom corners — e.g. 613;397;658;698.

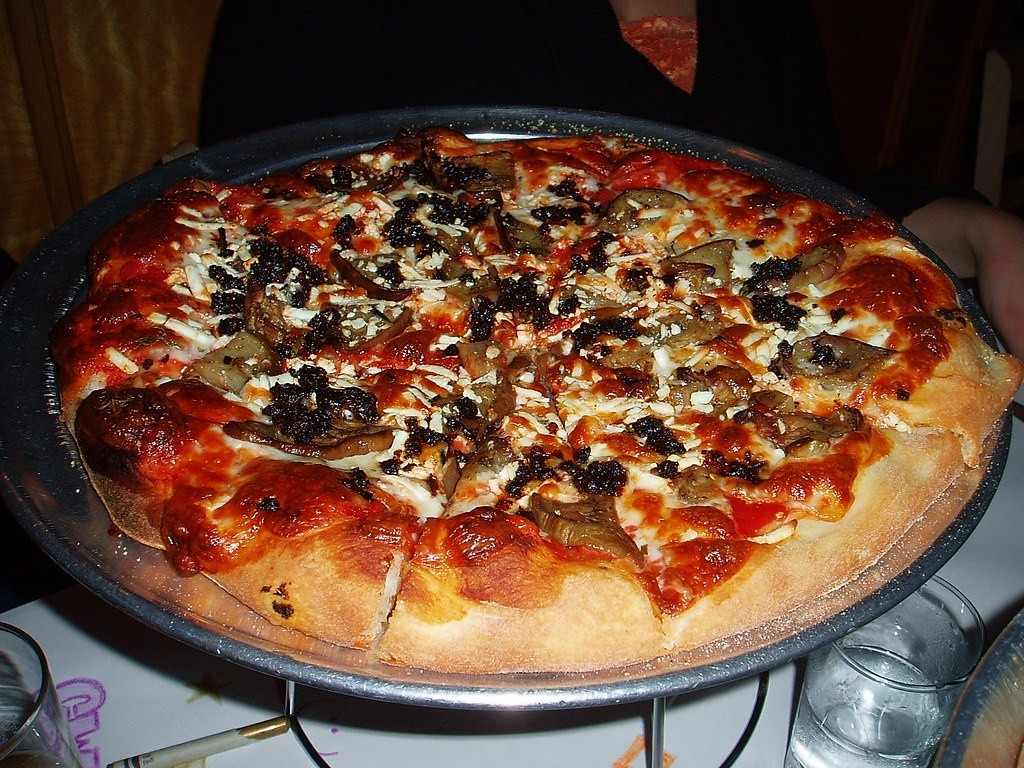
196;0;1023;370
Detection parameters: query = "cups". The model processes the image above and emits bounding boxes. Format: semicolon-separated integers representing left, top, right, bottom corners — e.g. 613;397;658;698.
784;577;985;768
0;622;81;768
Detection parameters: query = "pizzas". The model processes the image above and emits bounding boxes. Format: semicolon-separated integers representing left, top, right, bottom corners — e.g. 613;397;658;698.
51;125;1020;676
